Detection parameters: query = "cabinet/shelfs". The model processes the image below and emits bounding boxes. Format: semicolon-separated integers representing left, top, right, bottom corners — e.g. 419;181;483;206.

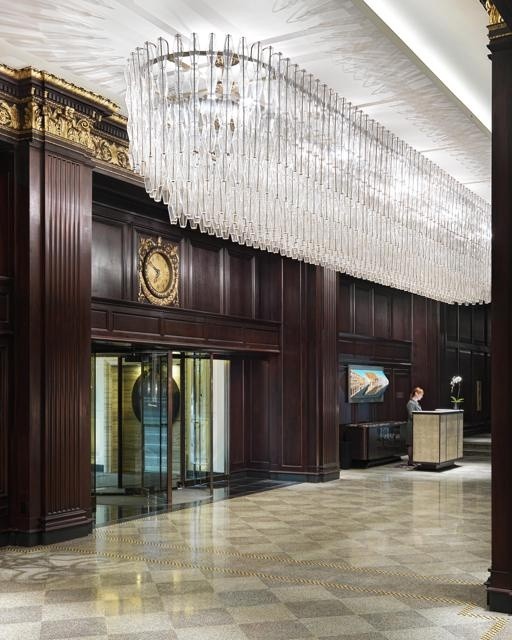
339;420;407;469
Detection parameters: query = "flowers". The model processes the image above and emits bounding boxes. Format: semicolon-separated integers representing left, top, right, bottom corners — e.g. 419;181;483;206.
450;376;464;403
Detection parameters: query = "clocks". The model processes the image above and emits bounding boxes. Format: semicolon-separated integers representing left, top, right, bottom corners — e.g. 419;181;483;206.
137;236;180;307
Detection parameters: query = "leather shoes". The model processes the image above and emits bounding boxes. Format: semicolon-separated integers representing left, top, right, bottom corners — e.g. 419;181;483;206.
407;461;415;466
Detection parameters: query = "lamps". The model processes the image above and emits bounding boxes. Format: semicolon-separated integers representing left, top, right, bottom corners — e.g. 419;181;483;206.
124;33;491;307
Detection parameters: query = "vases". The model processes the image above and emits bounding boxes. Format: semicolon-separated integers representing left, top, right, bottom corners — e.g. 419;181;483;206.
452;402;460;409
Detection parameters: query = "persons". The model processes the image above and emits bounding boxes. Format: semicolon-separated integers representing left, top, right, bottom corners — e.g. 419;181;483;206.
404;386;424;466
